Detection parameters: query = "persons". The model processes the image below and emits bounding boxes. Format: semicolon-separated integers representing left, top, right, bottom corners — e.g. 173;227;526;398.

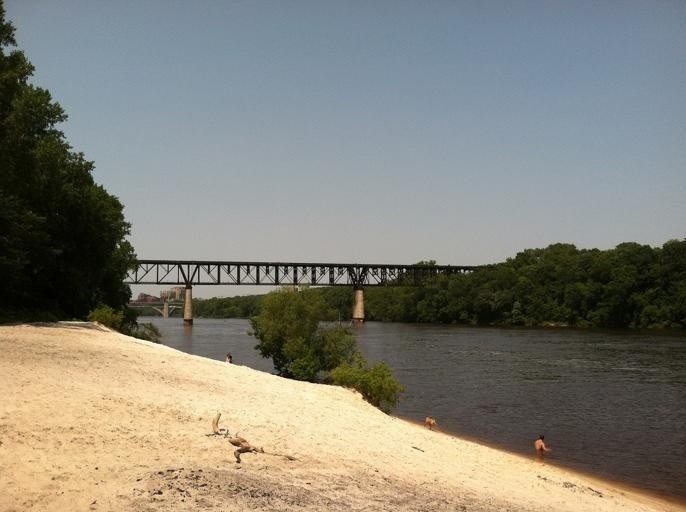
533;434;552;451
